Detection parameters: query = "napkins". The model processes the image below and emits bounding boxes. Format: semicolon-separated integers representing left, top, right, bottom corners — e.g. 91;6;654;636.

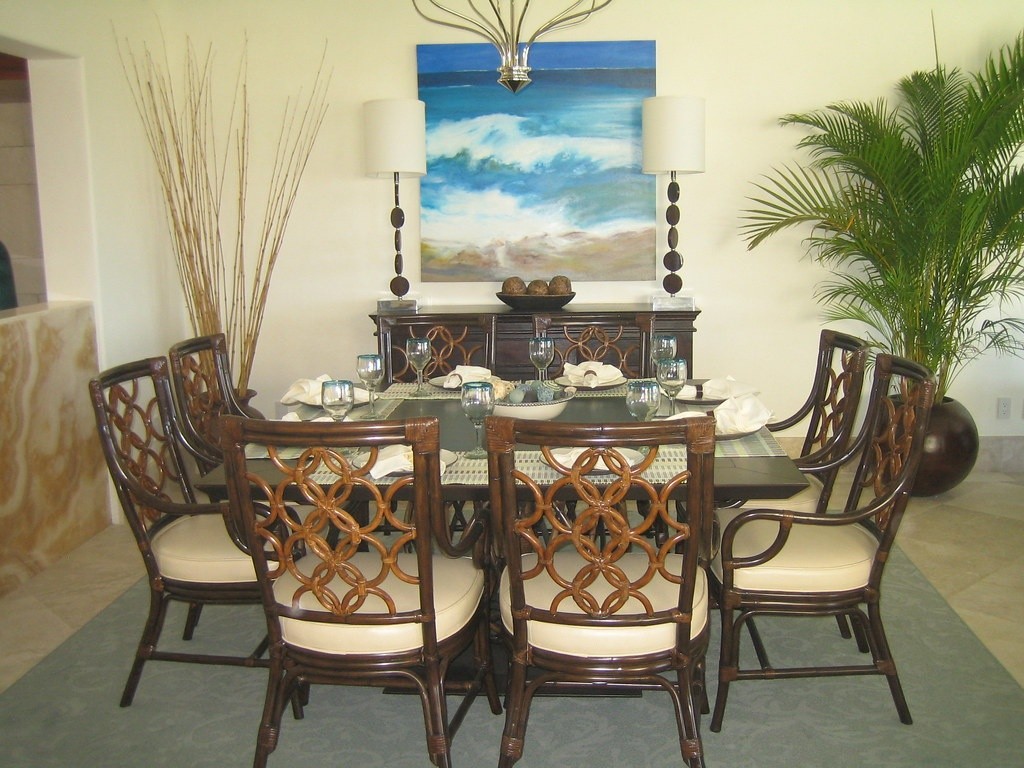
668;393;773;434
444;364;492;388
271;412;355;423
361;444;447;481
659;373;759;400
556;447;634;470
280;373;332;406
563;361;622;389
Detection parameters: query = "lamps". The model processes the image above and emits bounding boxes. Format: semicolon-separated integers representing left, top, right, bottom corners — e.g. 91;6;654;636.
640;95;706;311
362;96;427;314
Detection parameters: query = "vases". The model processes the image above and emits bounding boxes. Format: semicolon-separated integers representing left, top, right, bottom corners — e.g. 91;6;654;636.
198;387;265;422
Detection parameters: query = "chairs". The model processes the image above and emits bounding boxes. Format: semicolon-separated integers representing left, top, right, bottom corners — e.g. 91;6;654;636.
88;311;936;768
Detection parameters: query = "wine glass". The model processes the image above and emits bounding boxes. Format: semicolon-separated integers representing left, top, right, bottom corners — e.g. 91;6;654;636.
656;357;687;417
460;381;495;460
406;338;432;396
321;380;355;422
528;337;555;381
356;355;386;419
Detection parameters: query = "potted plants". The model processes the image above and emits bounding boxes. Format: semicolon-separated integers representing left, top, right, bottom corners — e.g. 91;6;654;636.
734;6;1024;494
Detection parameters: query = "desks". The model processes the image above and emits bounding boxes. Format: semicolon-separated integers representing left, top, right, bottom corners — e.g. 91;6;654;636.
191;380;811;713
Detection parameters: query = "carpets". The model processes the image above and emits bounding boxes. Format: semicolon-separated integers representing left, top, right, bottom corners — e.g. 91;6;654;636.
0;514;1024;768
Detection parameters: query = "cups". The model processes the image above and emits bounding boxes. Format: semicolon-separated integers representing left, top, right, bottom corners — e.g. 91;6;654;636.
649;334;678;365
625;380;661;422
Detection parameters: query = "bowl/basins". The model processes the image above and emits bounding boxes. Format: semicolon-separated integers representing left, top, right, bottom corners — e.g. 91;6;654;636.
496;291;576;311
493;393;574;420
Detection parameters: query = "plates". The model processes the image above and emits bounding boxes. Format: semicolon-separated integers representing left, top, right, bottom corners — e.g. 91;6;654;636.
428;375;501;389
298;388;379;408
352;448;458;477
539;446;646;475
666;410;762;441
555;376;628;389
676;396;724;407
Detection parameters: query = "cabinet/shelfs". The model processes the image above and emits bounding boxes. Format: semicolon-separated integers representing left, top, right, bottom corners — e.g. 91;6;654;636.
368;302;702;383
